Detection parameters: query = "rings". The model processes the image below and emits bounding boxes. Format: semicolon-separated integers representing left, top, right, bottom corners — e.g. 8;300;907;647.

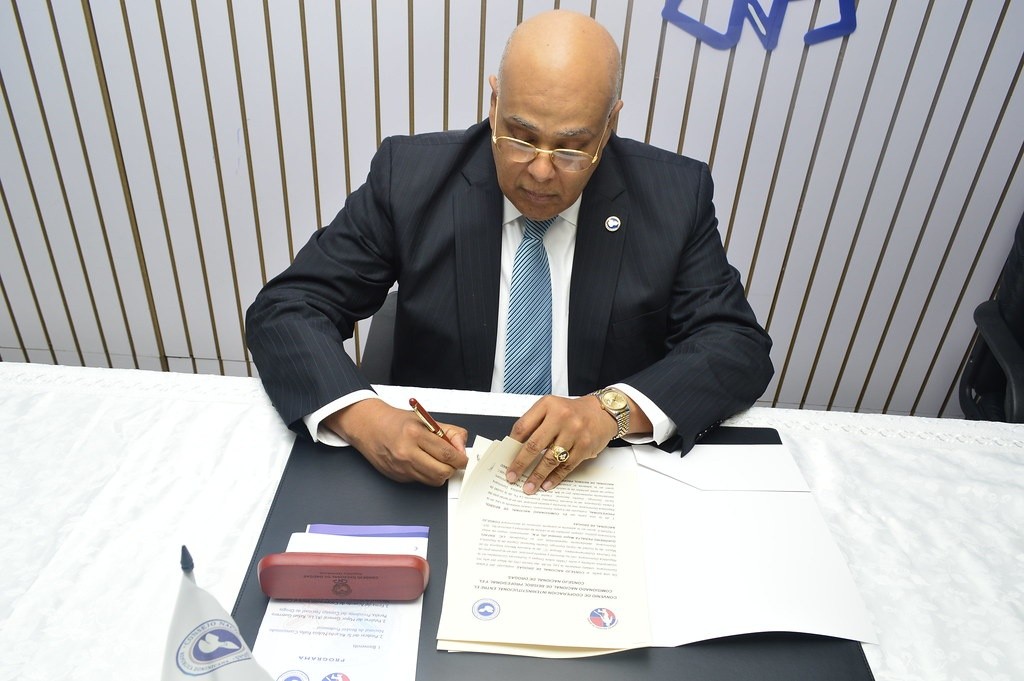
549;444;569;463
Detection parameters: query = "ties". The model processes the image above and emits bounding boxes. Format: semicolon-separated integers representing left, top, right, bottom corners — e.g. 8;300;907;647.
503;215;559;395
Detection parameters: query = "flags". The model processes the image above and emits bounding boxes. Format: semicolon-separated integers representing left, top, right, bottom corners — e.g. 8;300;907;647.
160;572;275;681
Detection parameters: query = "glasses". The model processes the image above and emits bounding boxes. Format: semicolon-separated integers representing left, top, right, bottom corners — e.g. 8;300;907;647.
493;96;610;173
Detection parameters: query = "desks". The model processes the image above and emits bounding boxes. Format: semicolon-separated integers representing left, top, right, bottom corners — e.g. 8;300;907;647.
0;361;1024;681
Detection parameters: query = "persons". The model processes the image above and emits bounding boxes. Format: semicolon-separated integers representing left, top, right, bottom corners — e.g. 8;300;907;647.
244;10;775;495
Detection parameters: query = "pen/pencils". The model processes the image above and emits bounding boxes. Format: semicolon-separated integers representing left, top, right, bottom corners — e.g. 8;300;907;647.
409;398;458;450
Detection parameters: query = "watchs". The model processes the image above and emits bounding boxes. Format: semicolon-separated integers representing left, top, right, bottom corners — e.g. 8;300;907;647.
587;389;630;440
